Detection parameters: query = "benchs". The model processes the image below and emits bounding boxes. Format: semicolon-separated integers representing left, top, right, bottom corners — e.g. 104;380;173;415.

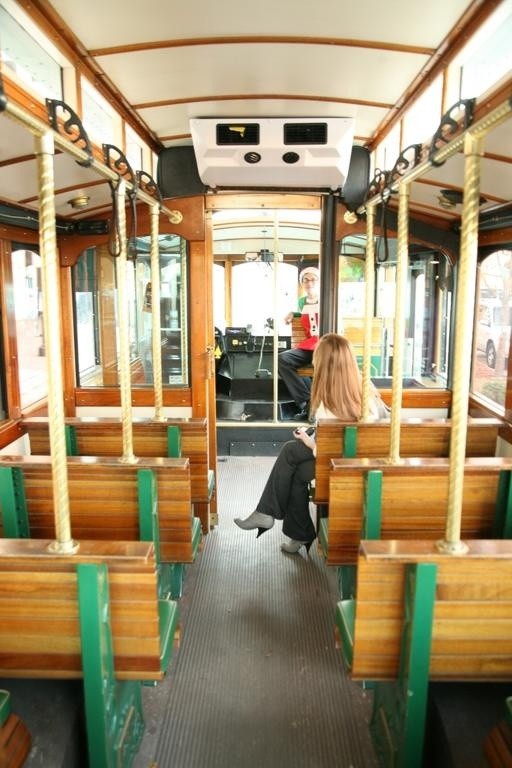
288;311;511;762
0;315;216;353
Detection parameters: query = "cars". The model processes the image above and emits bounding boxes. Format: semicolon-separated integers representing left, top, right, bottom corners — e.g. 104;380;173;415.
476;299;512;368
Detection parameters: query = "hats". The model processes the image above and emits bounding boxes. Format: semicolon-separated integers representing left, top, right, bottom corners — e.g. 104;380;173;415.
299;267;320;286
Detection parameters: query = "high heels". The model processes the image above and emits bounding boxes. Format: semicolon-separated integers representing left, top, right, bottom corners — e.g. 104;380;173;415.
281;532;316;554
234;509;274;538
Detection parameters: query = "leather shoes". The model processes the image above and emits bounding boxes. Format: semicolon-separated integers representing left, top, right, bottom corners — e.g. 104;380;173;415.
293;404;309;421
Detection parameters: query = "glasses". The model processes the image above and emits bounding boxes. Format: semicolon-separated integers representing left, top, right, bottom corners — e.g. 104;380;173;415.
302;278;319;283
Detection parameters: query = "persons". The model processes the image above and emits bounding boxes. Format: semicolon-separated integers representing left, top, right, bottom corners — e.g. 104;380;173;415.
234;333;391;554
278;268;320;421
